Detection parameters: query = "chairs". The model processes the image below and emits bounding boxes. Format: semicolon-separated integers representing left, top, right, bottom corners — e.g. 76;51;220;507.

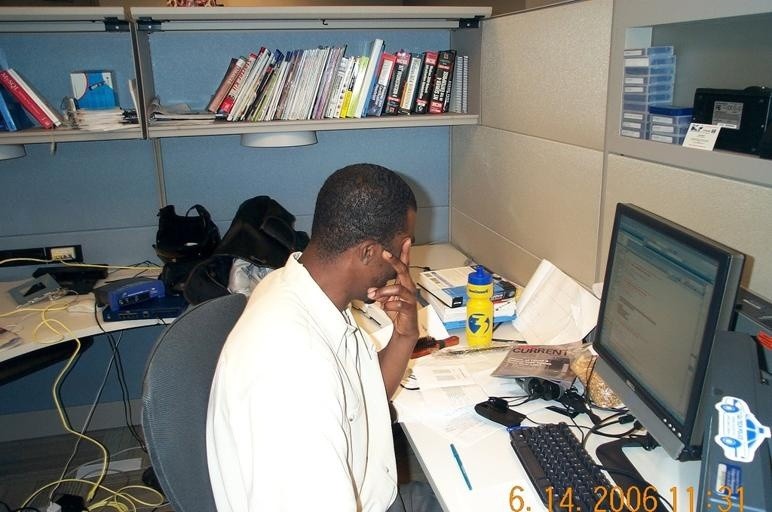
139;293;248;512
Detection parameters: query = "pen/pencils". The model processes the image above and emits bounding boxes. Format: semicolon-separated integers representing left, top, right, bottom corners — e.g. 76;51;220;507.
492;339;527;344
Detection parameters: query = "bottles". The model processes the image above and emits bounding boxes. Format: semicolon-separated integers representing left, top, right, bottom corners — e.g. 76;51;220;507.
465;266;494;348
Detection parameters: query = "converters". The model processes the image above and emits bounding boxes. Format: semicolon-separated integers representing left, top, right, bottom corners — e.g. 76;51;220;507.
56;493;84;512
46;503;62;512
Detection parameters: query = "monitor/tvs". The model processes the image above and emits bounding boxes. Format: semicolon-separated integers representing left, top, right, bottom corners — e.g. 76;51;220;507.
697;329;772;512
591;202;747;463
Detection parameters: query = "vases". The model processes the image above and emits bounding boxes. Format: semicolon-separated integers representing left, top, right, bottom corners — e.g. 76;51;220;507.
240;131;317;148
0;144;27;162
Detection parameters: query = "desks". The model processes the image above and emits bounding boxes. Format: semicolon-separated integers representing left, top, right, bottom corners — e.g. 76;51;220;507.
0;265;194;365
348;242;708;512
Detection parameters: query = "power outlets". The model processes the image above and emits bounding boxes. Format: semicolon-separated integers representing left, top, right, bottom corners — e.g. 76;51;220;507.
50;247;76;261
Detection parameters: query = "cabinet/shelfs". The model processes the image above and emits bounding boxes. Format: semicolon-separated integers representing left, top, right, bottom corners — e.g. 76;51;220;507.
0;7;145;145
129;6;494;140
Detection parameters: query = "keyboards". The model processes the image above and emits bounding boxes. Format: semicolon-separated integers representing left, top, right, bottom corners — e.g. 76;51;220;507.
508;422;630;512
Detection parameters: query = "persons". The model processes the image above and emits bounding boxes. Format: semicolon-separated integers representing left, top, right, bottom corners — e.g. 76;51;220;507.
207;163;443;512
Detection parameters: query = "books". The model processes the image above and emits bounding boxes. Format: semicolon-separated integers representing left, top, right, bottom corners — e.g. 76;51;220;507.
205;39;469;121
146;97;216;126
0;69;128;131
417;265;517;329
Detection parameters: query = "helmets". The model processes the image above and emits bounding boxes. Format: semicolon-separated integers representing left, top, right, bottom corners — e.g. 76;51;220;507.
153;195;310;305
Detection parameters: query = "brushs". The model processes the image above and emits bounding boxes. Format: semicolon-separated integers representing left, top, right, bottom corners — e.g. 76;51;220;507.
411;336;459;358
